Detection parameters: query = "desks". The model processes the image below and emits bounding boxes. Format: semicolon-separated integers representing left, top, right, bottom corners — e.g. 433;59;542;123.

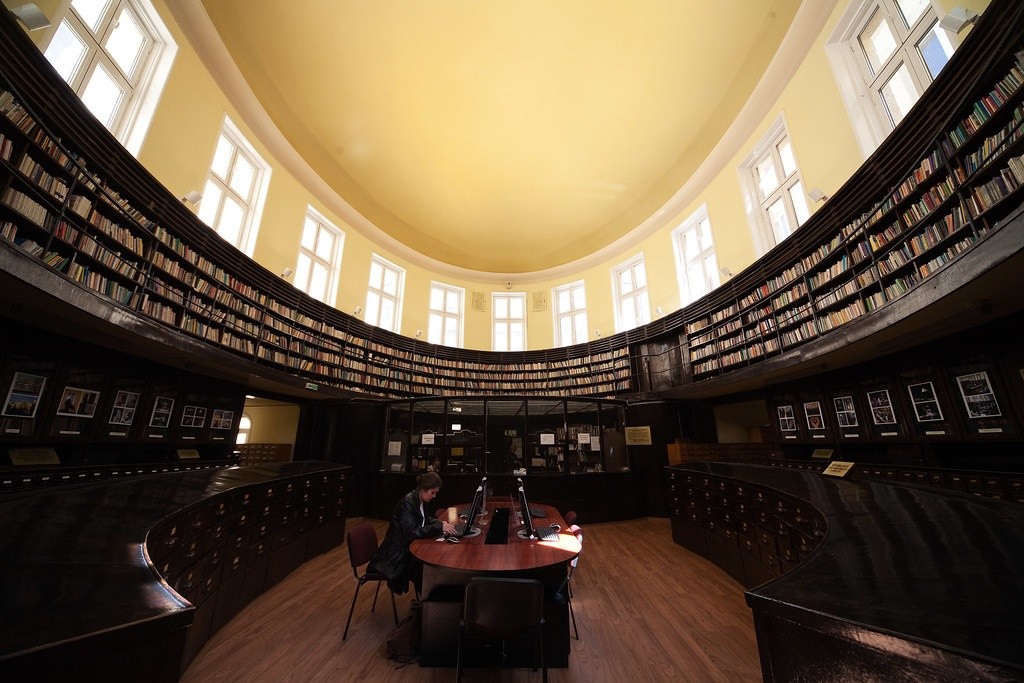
409;497;582;670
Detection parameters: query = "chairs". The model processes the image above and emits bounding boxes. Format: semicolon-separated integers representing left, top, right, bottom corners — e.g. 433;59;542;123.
342;523;419;640
568;524;584;640
565;511;577;527
455;577;548;683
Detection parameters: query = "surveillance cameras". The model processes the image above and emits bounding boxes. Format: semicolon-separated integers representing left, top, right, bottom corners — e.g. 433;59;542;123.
506;281;512;288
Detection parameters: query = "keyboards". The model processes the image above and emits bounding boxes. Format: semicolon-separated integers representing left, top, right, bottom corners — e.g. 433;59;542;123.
535;527;560;542
445;522;467;538
459;508;470;516
529;508;546;518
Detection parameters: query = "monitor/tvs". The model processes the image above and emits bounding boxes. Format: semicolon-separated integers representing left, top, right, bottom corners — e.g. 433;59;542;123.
463;477;488;535
518;477;538;537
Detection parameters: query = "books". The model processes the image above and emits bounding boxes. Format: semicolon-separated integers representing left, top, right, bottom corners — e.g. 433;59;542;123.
892;48;1024;279
686;262;817;374
413;354;502;399
258;299;413;401
801;193;920;333
571;346;630;396
0;90;155;306
138;226;266;355
502;360;571;396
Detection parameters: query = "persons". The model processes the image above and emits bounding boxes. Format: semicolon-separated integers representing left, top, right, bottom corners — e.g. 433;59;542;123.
427;457;440;472
372;472;457;598
504;444;518;471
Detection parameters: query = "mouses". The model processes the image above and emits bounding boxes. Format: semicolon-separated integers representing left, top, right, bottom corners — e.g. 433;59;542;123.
460;514;469;518
550;523;562;528
446;537;459;544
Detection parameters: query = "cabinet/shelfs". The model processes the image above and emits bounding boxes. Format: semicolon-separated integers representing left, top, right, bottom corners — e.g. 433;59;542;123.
0;0;1024;683
